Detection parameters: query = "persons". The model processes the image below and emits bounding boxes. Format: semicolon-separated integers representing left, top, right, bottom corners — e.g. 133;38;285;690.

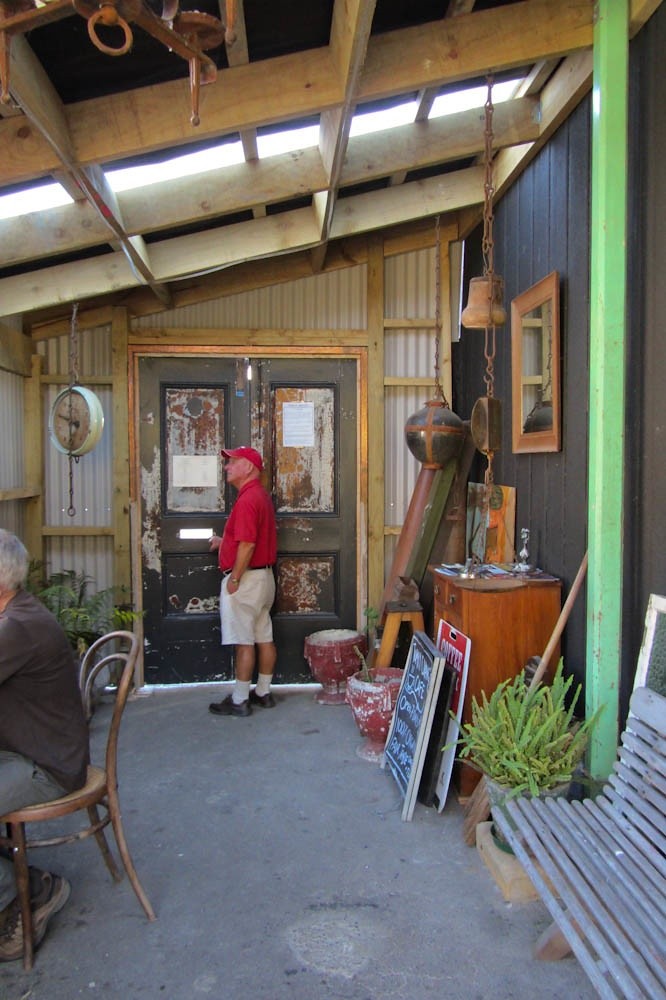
208;446;277;717
0;525;89;961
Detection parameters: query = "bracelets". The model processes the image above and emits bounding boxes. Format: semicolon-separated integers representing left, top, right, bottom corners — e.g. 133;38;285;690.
231;578;239;584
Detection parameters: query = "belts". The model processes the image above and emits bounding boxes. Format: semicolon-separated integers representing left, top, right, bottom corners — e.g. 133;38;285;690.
223;565;271;578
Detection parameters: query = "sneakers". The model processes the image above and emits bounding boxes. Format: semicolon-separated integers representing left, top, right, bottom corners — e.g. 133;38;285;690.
209;694;253;718
0;865;73;961
249;685;276;709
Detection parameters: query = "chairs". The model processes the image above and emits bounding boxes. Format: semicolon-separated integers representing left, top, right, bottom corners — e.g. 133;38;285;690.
0;629;156;971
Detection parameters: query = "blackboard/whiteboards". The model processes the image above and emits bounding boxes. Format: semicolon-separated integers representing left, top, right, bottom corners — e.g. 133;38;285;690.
384;631;446;801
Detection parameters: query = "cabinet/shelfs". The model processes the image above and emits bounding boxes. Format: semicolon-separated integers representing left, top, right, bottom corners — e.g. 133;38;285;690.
430;564;564;803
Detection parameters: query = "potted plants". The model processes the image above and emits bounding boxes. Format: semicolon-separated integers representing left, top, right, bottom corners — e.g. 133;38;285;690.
25;563;150;711
446;660;591;855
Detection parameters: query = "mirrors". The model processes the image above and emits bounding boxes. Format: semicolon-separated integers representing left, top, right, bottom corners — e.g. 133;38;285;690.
508;271;561;454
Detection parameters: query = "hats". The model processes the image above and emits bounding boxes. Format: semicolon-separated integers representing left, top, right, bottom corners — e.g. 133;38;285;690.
220;445;265;473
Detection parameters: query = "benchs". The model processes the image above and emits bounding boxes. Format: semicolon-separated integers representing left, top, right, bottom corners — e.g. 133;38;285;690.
490;686;666;1000
